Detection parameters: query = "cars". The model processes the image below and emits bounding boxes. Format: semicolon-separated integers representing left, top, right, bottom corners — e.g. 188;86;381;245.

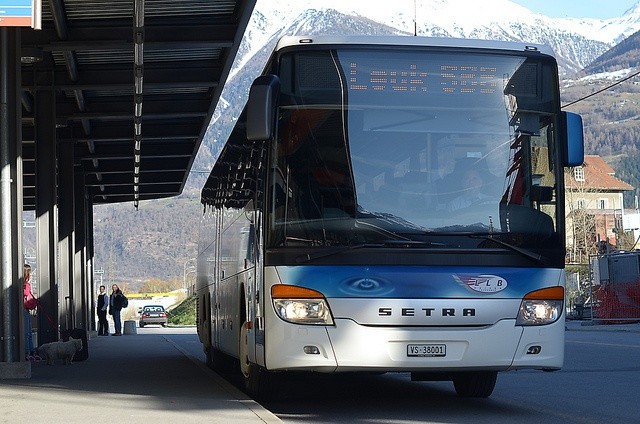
137;305;167;328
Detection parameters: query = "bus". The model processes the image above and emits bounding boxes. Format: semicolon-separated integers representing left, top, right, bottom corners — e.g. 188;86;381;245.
192;32;585;401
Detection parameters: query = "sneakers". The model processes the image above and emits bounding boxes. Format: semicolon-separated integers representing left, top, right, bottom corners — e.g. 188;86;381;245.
25;352;36;361
30;352;40;360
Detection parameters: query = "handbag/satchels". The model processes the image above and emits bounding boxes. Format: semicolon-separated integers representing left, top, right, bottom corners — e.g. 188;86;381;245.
116;293;128;307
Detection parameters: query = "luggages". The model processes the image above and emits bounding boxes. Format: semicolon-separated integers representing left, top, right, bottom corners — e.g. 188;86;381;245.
63;296;88;361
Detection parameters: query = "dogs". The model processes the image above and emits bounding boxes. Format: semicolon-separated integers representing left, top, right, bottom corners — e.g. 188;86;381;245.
37;336;83;366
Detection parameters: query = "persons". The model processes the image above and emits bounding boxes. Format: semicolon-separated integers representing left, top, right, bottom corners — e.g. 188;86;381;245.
109;284;123;336
449;172;492;214
23;264;42;361
97;285;108;336
